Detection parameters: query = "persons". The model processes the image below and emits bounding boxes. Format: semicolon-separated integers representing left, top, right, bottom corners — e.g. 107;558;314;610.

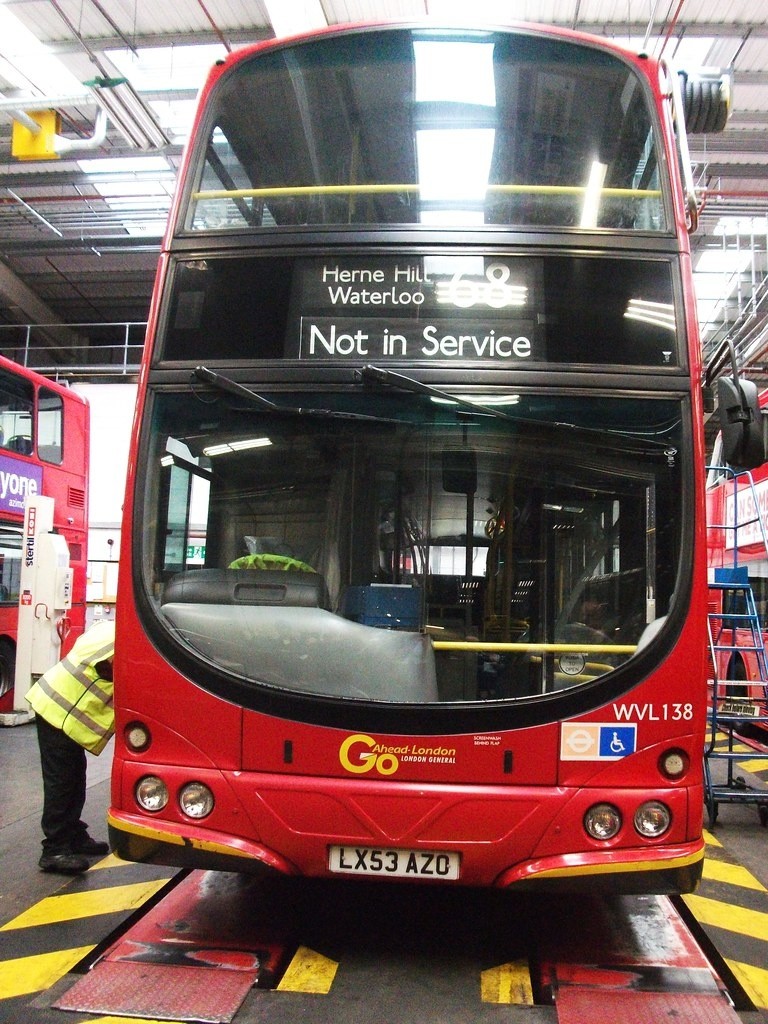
24;619;115;871
540;600;617;693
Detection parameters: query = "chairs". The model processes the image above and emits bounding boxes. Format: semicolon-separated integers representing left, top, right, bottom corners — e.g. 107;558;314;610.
245;537;323;568
0;425;61;464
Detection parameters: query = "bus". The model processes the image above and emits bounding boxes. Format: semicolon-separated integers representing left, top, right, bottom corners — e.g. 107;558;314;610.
705;385;768;751
0;354;91;731
108;18;727;901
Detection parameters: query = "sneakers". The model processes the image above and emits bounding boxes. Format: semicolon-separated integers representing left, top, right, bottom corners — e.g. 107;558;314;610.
38;828;111;876
